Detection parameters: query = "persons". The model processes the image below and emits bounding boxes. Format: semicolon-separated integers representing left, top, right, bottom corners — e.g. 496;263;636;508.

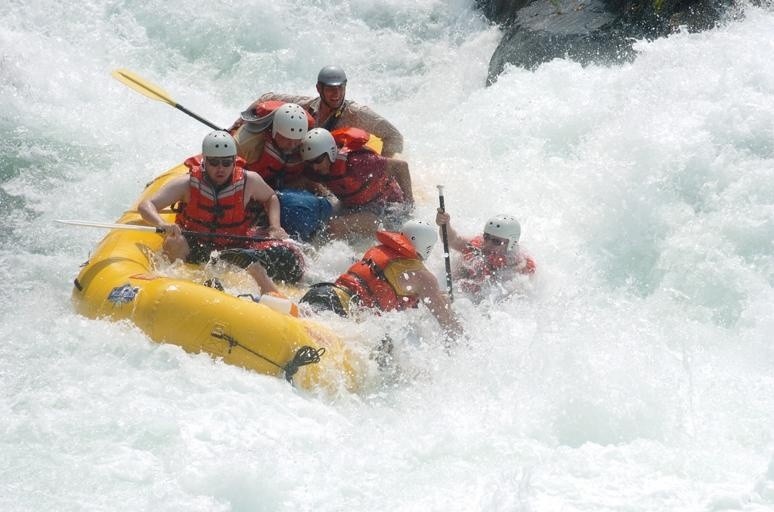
245;179;341;283
295;216;468;353
138;130;290;300
298;126;413;247
434;206;534;306
224;65;403;160
234;100;316;194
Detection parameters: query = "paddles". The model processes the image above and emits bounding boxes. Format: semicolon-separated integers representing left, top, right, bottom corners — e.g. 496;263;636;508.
115;67;228;133
52;218;282;242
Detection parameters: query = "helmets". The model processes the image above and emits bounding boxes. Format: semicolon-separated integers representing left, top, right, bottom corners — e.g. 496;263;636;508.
483;214;521;253
300;128;337;164
202;130;237;172
318;65;347;87
401;219;437;259
272;103;308;140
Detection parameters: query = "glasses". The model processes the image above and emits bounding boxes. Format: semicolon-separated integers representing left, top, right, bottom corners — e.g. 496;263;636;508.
304;153;327;167
206;159;233;167
483;233;508;245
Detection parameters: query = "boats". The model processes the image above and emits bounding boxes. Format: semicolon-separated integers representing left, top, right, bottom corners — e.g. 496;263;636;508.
69;123;430;399
